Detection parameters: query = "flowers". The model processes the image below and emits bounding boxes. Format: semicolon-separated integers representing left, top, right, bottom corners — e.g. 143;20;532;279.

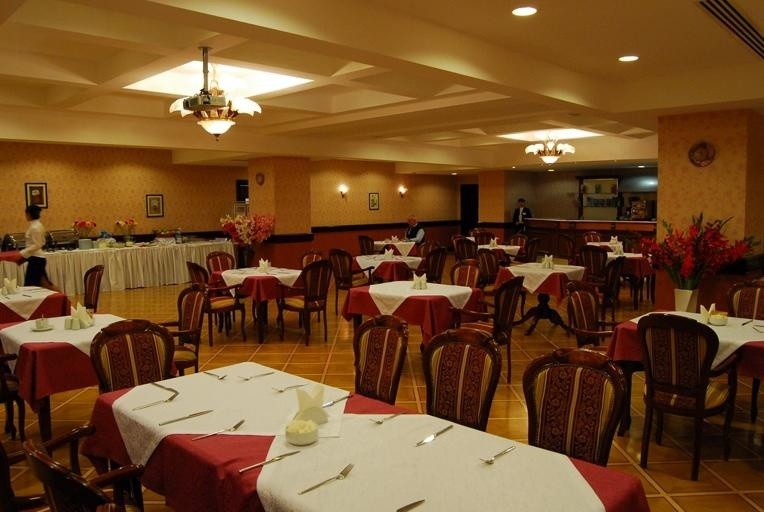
636;210;762;291
71;217;98;230
218;212;276;247
114;217;139;227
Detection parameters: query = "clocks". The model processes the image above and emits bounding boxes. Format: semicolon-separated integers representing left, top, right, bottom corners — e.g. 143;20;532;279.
688;141;715;167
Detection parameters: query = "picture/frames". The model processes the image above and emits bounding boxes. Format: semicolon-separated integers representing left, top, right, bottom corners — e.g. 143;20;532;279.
146;194;164;218
25;183;48;209
369;192;379;210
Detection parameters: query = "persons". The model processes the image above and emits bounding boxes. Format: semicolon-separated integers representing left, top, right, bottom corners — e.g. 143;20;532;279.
405;215;425;248
16;204;62;294
513;198;531;234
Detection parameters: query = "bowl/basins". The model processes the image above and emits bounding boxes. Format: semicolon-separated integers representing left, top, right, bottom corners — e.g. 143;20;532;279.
284;429;318;447
125;242;149;247
708;314;728;326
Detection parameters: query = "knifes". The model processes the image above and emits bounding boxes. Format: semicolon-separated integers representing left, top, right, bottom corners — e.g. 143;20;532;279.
159;410;212;426
321;393;352;408
238;450;302;472
395;500;427;512
416;425;451;446
151;380;179;394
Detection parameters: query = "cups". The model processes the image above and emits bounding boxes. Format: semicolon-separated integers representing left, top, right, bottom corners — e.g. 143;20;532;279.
35;319;48;328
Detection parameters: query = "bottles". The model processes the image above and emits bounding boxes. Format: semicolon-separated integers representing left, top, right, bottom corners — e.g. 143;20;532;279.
64;317;81;330
1;287;7;296
580;184;617;208
174;228;187;244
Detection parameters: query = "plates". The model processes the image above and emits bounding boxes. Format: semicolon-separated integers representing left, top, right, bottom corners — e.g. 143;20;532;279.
32;325;53;331
215;238;226;241
77;239;97;250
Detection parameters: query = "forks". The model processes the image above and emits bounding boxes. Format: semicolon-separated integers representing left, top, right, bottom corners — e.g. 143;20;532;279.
132;392;178;412
366;410;408;425
190;419;245;442
479;445;517;465
298;461;354;493
202;367;309;394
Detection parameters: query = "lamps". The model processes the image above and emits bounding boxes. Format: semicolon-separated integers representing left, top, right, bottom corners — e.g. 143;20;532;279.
169;64;262;142
524;135;576;166
